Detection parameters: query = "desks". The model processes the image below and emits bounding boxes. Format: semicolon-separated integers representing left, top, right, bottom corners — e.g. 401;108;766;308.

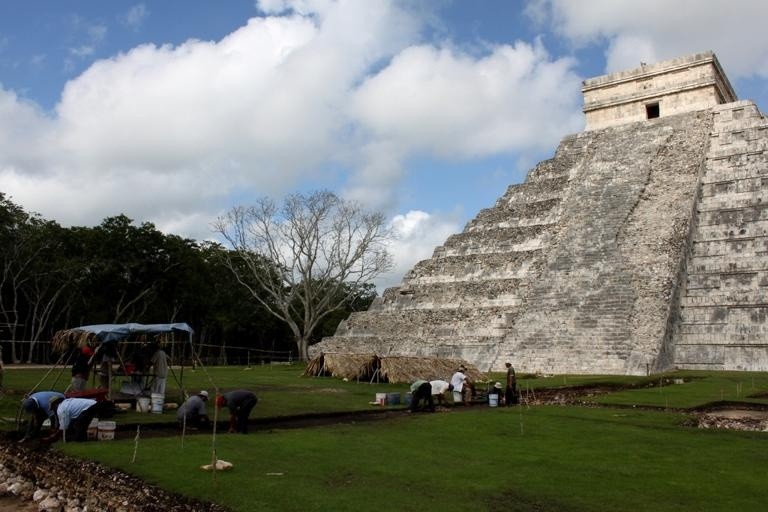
93;366;158;402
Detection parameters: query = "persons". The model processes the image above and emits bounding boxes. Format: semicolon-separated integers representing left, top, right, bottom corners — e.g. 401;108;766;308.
487;382;505;408
504;362;517;405
20;391;66;441
214;389;257;435
46;395;98;443
71;344;170;396
429;379;454;407
176;390;212;434
405;380;435;414
449;368;472;408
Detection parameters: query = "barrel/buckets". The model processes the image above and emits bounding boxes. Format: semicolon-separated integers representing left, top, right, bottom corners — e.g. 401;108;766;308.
97;421;116;440
390;393;400;402
137;397;150;412
405;392;413;403
86;417;98;438
386;394;392;404
376;392;386;405
151;393;164;414
489;393;498;407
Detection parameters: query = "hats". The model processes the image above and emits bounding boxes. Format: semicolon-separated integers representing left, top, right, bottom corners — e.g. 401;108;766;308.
200;391;210;400
218;396;222;406
23;399;35;413
48;397;58;411
